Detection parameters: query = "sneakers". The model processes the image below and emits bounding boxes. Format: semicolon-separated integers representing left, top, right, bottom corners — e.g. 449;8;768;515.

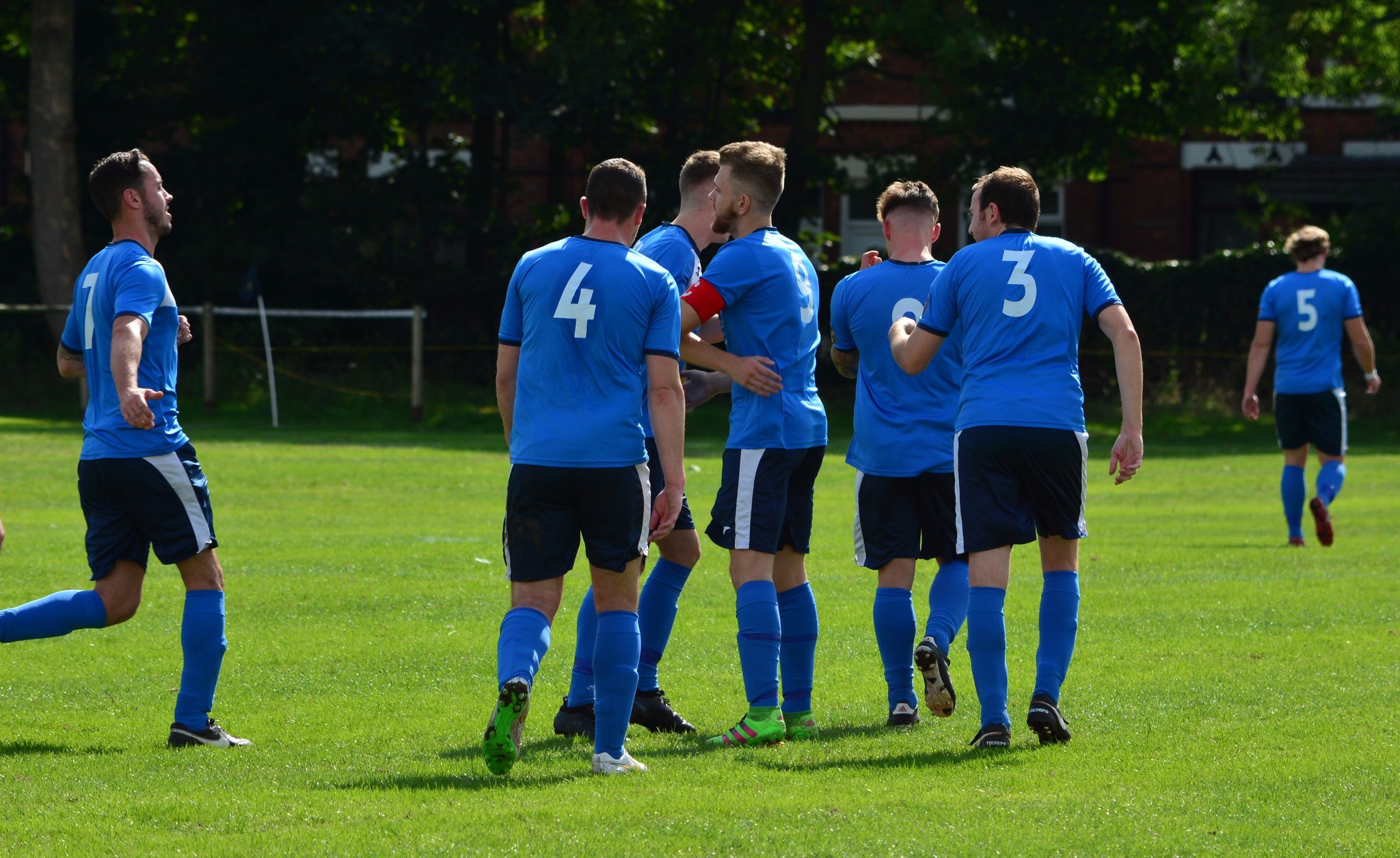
970;723;1011;748
483;677;530;775
887;703;920;725
914;636;956;717
167;718;252;750
1027;694;1071;745
629;687;696;733
553;696;595;739
783;714;820;739
704;709;786;747
592;747;647;775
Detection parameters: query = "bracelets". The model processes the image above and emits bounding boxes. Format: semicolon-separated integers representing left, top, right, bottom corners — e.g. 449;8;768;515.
1365;368;1377;381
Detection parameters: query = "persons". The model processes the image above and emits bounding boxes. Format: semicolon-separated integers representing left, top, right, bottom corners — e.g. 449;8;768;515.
1242;226;1382;546
680;142;829;748
830;180;970;725
888;164;1144;747
0;149;252;748
553;149;784;738
481;158;688;777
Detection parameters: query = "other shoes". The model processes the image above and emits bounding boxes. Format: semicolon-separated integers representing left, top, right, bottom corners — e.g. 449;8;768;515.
1310;497;1333;546
1288;536;1306;546
